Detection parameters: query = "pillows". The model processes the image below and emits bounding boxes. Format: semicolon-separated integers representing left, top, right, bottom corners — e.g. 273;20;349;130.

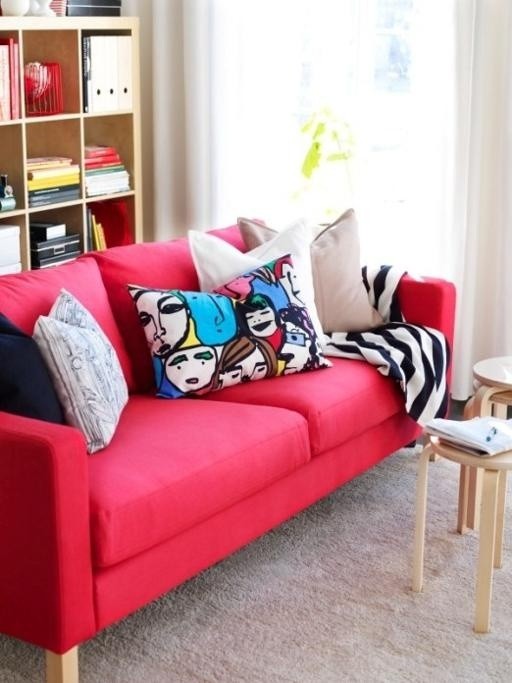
186;221;326;343
32;286;128;456
236;206;388;335
1;311;64;426
126;251;334;400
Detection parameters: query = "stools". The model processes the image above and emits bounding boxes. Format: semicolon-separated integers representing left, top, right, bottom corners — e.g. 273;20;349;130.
410;355;512;634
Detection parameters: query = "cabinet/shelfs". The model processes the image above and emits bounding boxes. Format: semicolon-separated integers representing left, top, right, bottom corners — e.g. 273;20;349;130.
0;16;139;279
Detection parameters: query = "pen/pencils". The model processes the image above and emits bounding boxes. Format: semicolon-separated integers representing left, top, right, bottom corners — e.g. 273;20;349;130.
486;426;497;441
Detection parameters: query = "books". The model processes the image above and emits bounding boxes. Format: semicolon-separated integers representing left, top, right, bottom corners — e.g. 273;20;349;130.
26;154;83;208
30;221;78;268
0;38;20;124
84;145;129;196
86;205;106;249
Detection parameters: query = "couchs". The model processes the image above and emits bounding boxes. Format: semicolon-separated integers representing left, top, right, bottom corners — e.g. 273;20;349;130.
1;220;457;683
